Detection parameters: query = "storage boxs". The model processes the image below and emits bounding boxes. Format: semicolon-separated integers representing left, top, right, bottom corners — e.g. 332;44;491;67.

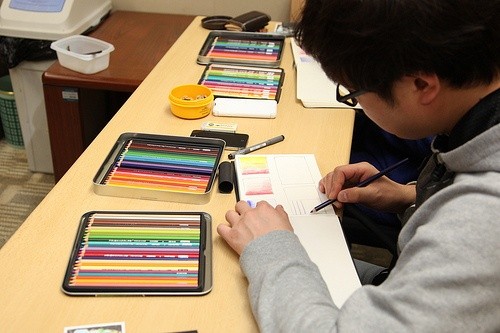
50;33;115;74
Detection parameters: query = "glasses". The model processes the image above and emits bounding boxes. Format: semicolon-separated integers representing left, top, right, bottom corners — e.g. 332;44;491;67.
335;83;370;107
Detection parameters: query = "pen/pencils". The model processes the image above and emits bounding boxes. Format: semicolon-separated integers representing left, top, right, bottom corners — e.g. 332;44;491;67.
201;37;283;99
70;213;200;287
171;330;197;333
310;157;409;213
228;135;284;159
104;140;219;192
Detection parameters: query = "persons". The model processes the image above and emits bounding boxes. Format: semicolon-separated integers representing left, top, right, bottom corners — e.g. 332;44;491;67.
216;0;500;333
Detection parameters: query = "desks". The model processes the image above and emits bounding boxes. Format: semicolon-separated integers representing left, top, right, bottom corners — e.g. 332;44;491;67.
0;13;357;333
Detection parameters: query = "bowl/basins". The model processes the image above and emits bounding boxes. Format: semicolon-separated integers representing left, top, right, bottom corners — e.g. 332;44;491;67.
168;84;214;119
50;35;115;74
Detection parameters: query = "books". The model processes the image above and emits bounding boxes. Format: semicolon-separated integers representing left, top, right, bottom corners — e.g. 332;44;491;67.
230;153;363;309
288;37;364;110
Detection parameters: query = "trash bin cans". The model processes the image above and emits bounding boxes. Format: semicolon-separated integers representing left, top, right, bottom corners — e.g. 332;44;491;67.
0;75;24;149
